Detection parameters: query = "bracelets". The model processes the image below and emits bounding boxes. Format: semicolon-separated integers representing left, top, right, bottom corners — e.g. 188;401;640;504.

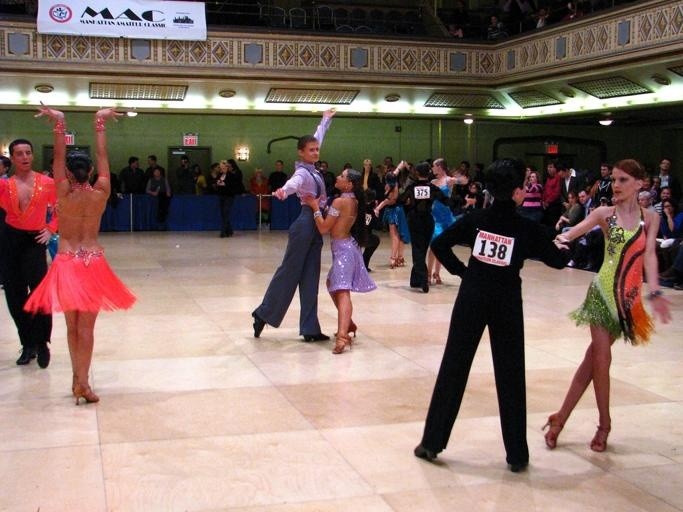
94;117;108;130
313;210;322;219
645;289;663;302
50;120;66;135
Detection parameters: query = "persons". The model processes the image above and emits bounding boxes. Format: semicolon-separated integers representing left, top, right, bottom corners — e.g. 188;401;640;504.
252;107;337;342
1;154;288;239
414;158;575;472
303;168;378;355
22;100;137;405
541;158;679;452
315;157;494;294
0;139;59;369
519;153;682;292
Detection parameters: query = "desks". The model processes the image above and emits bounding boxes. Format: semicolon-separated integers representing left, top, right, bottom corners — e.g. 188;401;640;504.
270;194;304;227
98;198;258;230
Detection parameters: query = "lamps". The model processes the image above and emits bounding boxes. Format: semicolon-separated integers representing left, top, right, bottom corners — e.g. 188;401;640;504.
548;144;559;154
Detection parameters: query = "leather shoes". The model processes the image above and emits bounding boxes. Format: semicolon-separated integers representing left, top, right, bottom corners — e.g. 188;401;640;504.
420;281;429;293
16;344;37;364
659;268;679;280
36;340;50;368
252;312;268;337
413;444;436;460
305;333;330;342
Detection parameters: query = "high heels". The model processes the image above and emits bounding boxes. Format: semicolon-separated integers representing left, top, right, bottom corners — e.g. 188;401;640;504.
389;254;443;285
333;334;352;353
334;319;357;337
74;382;99;405
541;414;564;449
590;424;611;452
